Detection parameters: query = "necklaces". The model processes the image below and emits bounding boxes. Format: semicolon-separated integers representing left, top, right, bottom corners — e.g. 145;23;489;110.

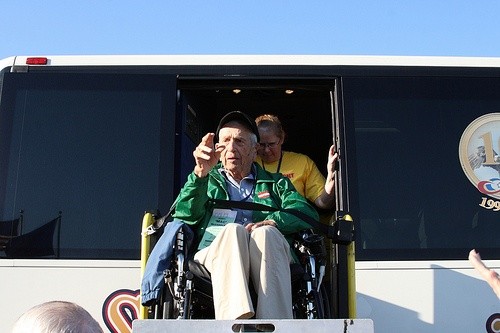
261;154;283;174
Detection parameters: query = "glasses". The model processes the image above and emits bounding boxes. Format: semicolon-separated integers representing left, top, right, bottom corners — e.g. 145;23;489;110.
257;139;281;146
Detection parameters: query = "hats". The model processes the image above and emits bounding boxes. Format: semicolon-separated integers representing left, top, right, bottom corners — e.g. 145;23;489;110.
216;110;260;142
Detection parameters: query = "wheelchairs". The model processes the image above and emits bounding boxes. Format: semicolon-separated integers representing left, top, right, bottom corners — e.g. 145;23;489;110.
153;230;335;319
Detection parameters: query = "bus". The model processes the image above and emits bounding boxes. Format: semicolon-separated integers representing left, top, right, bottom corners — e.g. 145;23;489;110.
0;53;500;333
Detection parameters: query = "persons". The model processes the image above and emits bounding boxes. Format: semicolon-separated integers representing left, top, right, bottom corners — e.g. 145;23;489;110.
10;301;104;333
170;110;321;320
253;114;338;209
